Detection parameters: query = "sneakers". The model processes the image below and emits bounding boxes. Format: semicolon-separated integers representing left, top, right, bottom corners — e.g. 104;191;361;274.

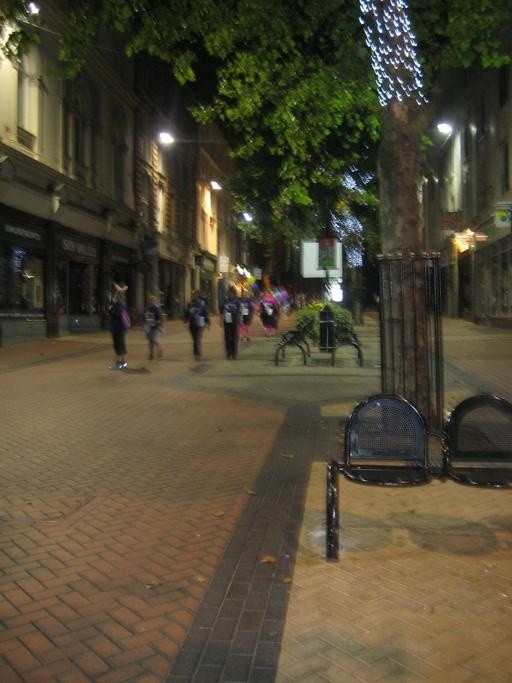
148;348;163;361
226;352;238;361
108;361;128;370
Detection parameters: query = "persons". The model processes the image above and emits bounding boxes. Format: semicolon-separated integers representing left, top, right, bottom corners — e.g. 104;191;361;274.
235;286;306;342
219;286;242;360
184;289;211;362
141;294;163;360
108;287;131;370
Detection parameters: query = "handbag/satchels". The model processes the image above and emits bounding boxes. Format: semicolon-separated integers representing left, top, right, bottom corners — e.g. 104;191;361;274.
121;310;131;328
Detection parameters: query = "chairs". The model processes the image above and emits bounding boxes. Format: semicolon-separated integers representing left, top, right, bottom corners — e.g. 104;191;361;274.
273;304;365;367
441;391;510;489
324;392;448;561
